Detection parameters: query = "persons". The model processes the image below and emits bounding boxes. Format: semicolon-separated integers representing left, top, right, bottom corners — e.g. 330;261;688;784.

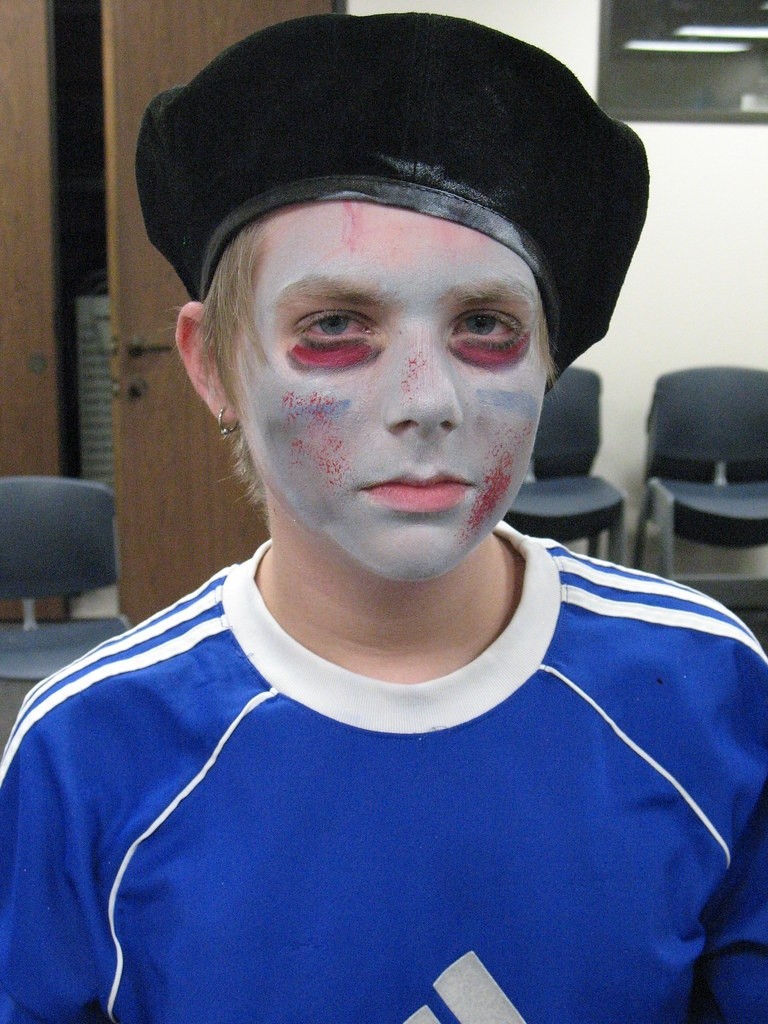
2;16;768;1024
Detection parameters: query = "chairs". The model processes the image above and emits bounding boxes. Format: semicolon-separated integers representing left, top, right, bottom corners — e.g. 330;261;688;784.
503;367;768;611
0;476;130;682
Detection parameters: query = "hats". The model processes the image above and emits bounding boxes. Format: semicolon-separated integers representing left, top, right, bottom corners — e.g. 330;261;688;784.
135;12;650;394
